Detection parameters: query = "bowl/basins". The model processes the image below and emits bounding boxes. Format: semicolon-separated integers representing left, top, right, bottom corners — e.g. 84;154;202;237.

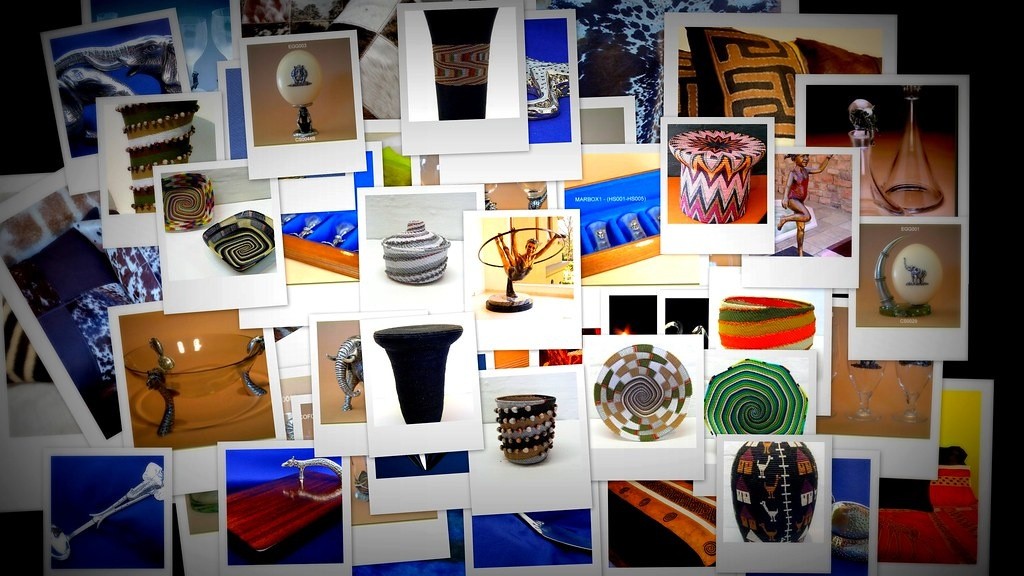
123;335;259;399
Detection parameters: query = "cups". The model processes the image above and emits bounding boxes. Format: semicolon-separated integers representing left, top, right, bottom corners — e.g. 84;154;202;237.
494;393;558;465
382;219;451;285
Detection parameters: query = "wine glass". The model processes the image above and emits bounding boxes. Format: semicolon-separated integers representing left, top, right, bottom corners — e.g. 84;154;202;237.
895;360;935;424
846;346;887;423
817;320;843;419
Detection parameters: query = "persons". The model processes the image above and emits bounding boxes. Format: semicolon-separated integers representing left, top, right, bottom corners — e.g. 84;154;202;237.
494;227;566;297
777;154;833;256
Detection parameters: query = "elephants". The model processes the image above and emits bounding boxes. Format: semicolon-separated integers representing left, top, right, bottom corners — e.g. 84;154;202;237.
54;35;182;94
57;68;137;147
326;334;364;412
903;258;927;285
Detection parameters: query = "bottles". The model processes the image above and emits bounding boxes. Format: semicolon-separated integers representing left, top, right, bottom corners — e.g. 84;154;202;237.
848;85;944;216
